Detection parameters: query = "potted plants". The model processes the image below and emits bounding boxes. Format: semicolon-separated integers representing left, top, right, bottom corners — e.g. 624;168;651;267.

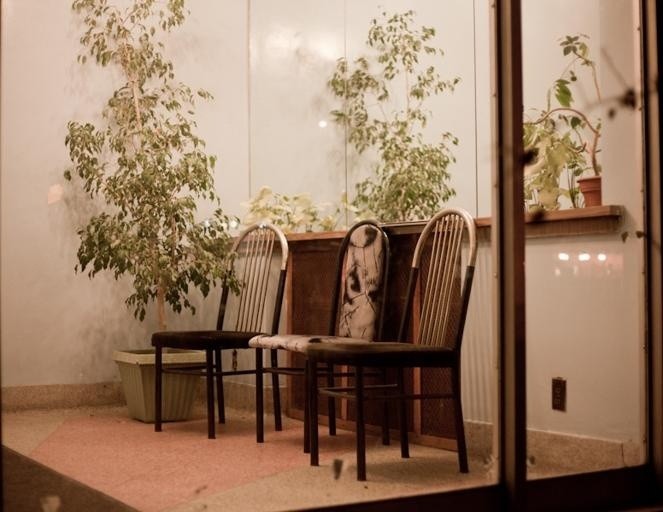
522;32;600;206
62;1;248;422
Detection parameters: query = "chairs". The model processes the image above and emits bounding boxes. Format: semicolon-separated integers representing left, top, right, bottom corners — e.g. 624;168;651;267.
308;206;479;480
151;222;290;439
249;218;391;453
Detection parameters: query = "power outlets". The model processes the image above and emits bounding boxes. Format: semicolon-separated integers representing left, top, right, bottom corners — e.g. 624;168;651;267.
552;378;566;411
